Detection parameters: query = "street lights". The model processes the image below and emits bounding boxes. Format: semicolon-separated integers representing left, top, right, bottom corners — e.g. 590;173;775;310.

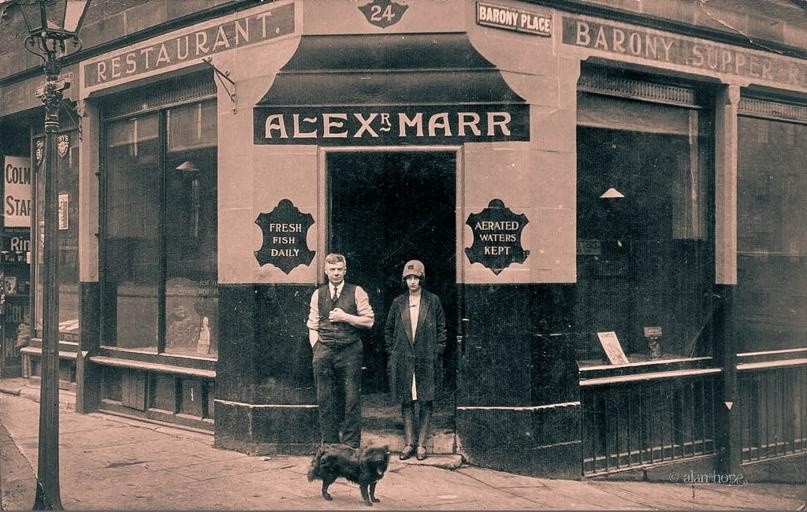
15;0;93;512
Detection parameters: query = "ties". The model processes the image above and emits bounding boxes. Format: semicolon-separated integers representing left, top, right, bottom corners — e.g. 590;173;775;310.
332;288;338;303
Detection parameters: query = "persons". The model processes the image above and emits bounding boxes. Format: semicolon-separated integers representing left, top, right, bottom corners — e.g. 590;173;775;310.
384;260;446;460
307;254;375;447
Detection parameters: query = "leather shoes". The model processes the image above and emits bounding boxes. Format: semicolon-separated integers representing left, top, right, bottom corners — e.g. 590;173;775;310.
399;444;427;460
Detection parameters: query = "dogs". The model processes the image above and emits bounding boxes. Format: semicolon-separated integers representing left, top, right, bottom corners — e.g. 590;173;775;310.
306;443;390;507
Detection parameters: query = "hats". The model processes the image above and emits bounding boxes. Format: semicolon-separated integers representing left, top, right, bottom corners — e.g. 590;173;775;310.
403;260;425;278
